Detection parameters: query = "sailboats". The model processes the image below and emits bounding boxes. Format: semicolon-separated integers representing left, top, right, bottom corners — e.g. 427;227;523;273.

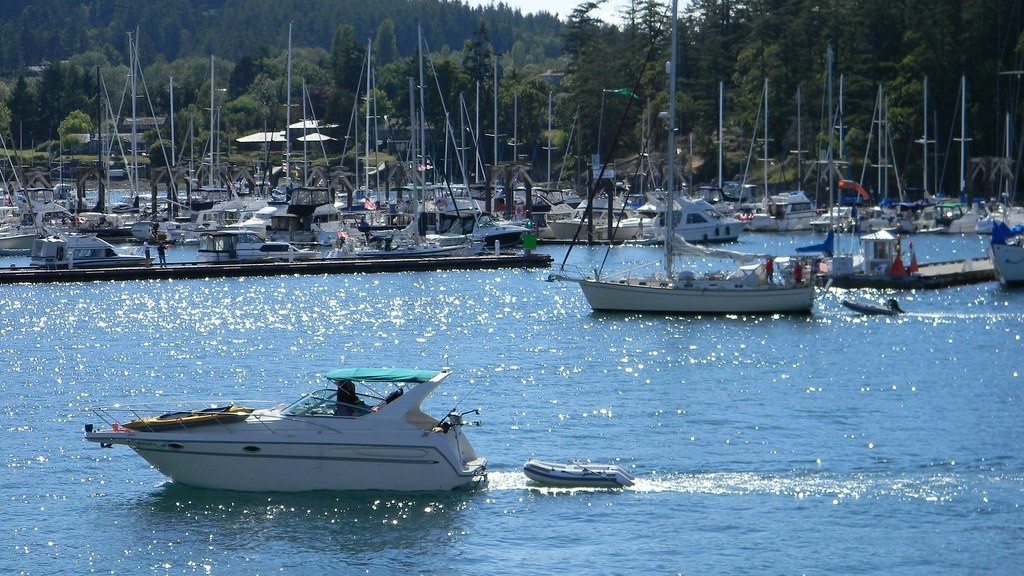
0;21;1024;290
546;0;838;318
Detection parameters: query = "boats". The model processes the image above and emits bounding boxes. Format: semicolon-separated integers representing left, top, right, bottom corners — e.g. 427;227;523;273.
521;460;636;490
84;366;487;493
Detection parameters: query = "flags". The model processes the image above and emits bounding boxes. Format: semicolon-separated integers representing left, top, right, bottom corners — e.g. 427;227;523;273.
604;88;638;98
623;178;632;196
365;199;377;210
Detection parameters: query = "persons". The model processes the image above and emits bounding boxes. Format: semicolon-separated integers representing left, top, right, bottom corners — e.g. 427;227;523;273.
638;218;644;240
143;237;151;261
795;262;803;283
3;194;9;206
766;258;774;284
157;242;167;267
333;380;372;417
